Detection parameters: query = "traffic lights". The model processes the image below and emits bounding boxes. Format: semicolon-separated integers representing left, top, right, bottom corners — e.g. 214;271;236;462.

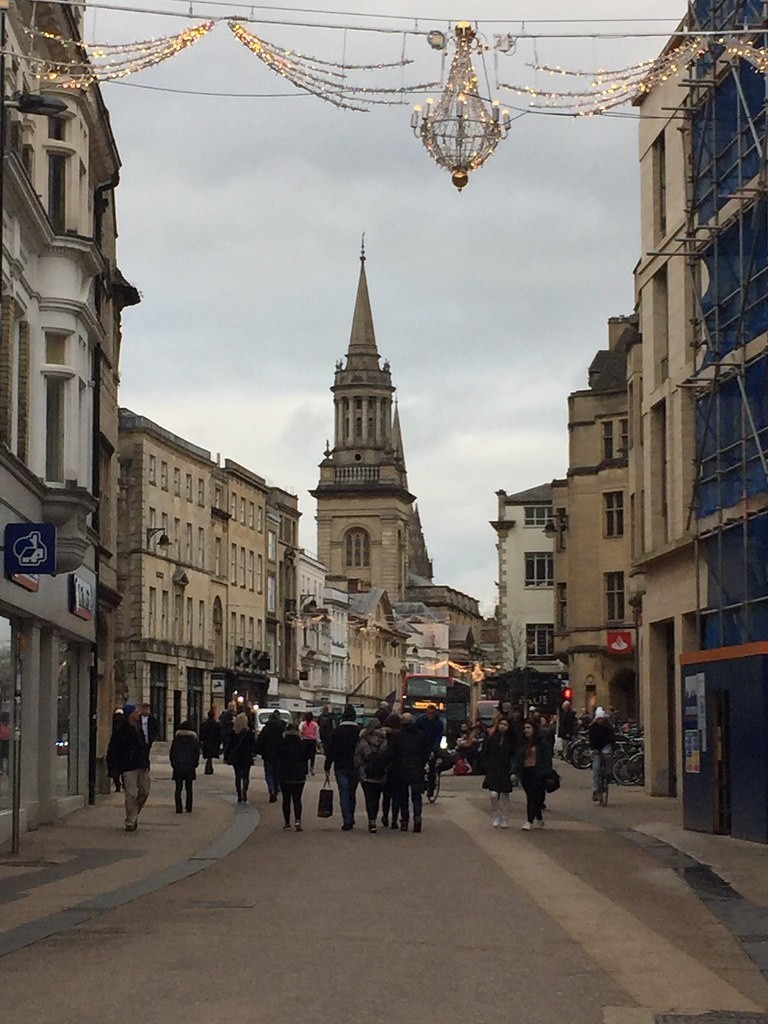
563;687;573;701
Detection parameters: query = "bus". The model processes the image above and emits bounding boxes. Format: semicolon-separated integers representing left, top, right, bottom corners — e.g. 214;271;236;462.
403;671;556;737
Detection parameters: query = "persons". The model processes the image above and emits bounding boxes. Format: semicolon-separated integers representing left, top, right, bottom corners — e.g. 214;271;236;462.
107;704;152;832
482;719;520;828
199;700;289;803
456;705;557;785
589;706;615;805
299;701;444;834
558;700;578;760
136;703;158;773
511;720;554;831
278;723;314;832
169;720;199;813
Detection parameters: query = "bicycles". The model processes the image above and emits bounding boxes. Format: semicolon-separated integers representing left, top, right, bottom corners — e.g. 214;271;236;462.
424;761;440;802
563;719;645;786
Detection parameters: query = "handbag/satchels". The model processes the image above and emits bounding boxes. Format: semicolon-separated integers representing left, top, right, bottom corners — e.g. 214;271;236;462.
544;770;560;793
318;775;333;817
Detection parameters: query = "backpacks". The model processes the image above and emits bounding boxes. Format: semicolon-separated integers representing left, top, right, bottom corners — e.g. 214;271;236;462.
365;739;387;779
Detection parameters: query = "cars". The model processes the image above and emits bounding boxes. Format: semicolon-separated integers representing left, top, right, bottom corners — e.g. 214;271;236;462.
256;708;297;739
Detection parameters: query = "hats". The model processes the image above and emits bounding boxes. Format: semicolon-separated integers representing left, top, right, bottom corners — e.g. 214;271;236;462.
123;704;136;718
380;701;388;708
181;721;191;729
384;714;400;728
595;706;605;719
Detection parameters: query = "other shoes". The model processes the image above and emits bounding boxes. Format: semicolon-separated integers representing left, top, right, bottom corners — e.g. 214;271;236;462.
502;816;509;828
295;820;303;831
270;795;277;802
522;821;532;830
243;793;246;802
126;823;138;831
392;821;399;829
283;823;291;830
342;823;351;830
238;794;242;802
382;816;388;827
413;823;422;832
493;816;502;827
370;825;377;833
534;820;545;828
400;819;408;831
